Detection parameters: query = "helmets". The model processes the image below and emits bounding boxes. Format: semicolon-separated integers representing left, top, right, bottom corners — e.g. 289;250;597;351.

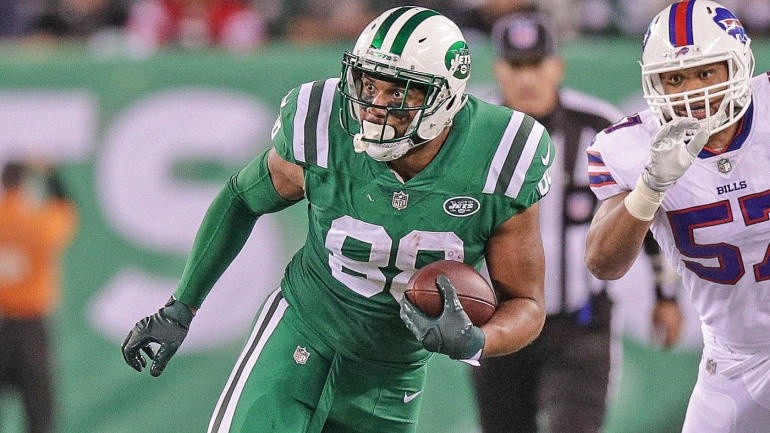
637;0;755;137
337;7;471;162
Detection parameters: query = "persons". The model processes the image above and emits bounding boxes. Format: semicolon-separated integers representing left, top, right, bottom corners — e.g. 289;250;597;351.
583;0;768;432
0;153;86;433
117;4;558;433
470;11;688;432
22;1;766;53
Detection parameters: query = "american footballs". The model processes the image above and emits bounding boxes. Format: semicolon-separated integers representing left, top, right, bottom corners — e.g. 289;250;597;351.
405;260;495;326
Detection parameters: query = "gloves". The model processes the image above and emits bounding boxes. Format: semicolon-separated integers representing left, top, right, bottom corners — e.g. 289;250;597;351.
121;296;195;376
625;119;709;221
398;273;485;368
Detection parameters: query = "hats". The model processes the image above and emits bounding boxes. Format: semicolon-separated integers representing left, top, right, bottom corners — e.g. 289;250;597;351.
493;11;566;62
